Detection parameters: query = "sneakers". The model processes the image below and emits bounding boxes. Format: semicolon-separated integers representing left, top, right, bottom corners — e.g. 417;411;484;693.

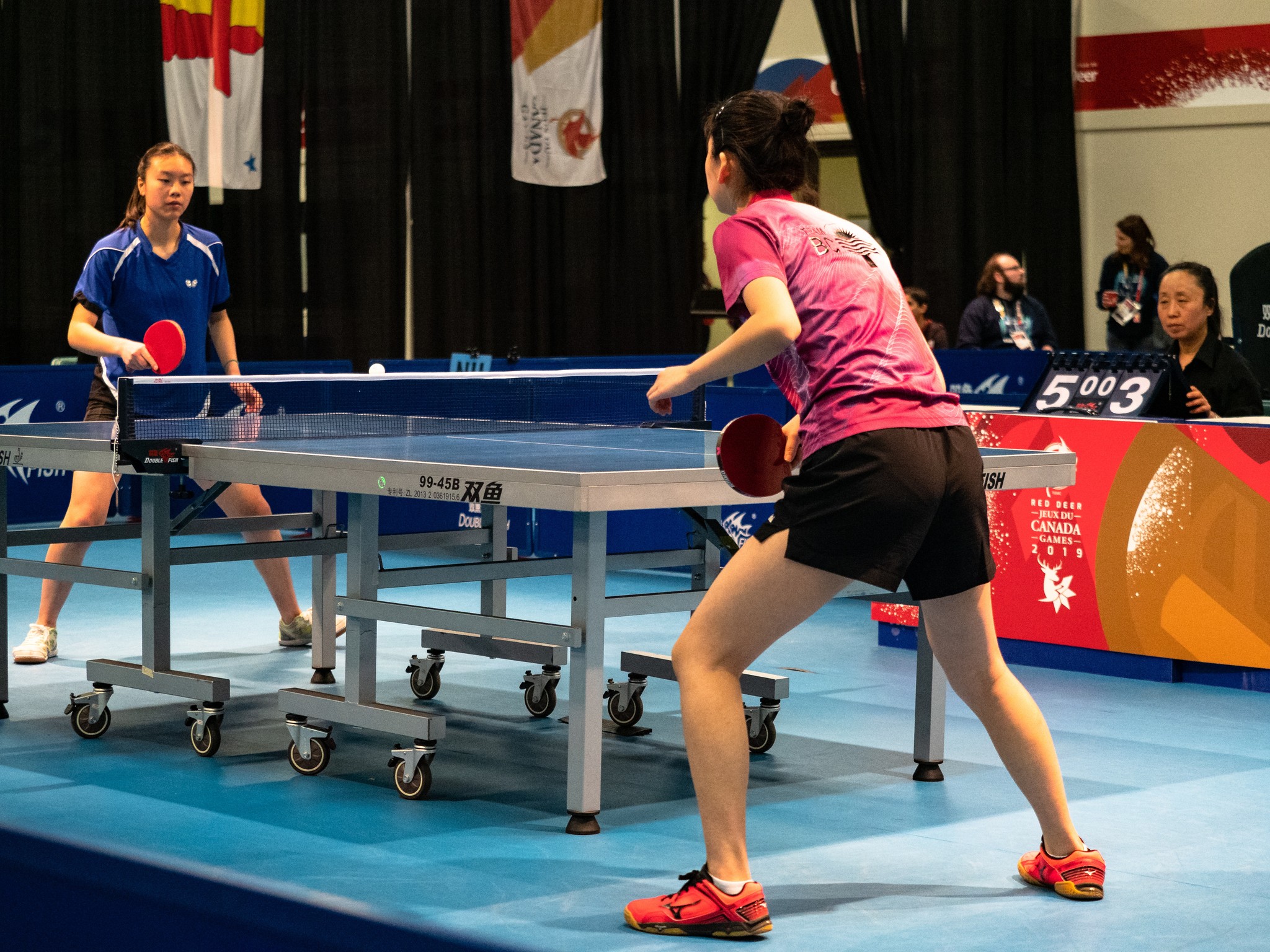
623;863;772;937
12;623;59;663
1018;834;1105;901
278;608;347;646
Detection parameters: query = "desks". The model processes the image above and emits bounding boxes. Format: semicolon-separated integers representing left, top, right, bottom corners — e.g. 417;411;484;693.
870;399;1270;693
0;414;1076;835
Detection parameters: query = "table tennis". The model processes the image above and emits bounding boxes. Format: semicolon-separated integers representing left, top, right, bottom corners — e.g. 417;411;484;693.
369;363;386;374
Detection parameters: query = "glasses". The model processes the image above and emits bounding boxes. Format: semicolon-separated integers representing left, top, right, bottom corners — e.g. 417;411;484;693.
1000;265;1022;273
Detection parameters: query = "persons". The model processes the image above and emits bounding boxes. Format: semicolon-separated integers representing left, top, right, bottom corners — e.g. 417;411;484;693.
905;287;948;351
1230;243;1270;398
1095;213;1169;353
12;142;348;667
1153;262;1264;418
623;90;1105;937
957;253;1057;354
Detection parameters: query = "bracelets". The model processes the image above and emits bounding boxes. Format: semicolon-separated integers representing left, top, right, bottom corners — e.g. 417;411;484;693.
1214;412;1219;418
223;359;238;370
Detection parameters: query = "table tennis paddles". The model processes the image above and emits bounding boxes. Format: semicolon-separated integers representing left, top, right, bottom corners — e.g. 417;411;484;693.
123;316;186;378
715;412;791;499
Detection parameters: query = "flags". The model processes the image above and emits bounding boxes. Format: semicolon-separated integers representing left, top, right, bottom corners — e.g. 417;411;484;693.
510;0;607;187
159;0;266;191
